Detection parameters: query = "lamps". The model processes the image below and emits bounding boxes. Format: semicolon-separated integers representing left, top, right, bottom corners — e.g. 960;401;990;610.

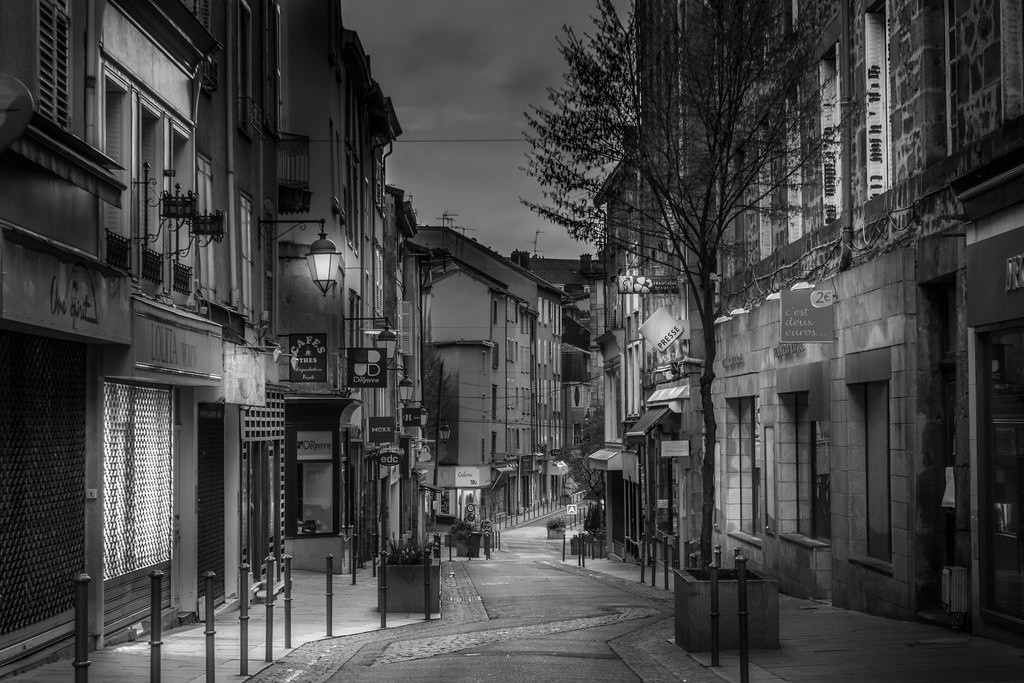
387;367;415;408
262;352;300;371
428;418;451;446
343;314;398;368
396;401;430;431
234;344;282;363
257;215;342;297
